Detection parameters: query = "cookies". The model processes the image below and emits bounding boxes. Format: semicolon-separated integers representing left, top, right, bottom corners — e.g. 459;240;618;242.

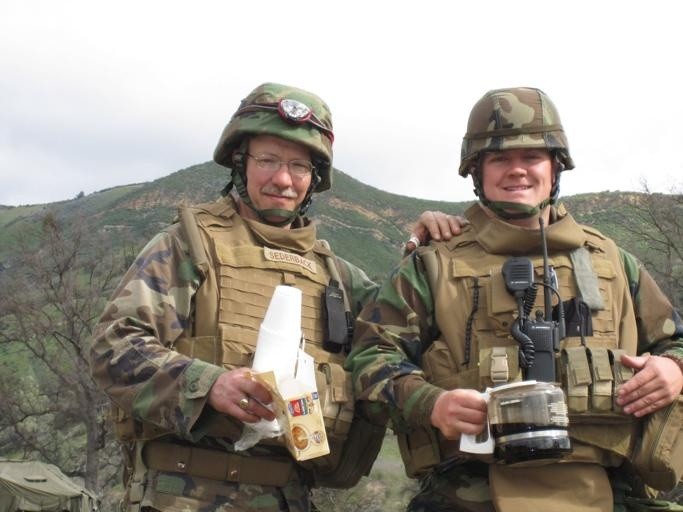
293;427;308;450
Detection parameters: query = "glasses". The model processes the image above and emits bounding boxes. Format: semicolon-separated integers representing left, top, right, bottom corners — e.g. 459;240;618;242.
246;151;316;176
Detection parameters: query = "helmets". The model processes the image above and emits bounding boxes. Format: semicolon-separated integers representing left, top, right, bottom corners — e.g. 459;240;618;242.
460;87;575;177
214;82;333;192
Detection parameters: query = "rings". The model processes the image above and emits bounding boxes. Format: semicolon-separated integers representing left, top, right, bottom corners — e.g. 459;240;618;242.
239;397;249;410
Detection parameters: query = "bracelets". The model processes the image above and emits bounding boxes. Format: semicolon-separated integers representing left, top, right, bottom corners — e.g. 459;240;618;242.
660;353;683;396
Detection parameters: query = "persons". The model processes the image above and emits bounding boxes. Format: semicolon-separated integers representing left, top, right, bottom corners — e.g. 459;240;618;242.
89;83;468;512
344;88;683;512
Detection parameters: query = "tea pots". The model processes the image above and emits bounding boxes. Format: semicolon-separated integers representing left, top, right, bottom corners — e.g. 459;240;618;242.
456;378;573;469
